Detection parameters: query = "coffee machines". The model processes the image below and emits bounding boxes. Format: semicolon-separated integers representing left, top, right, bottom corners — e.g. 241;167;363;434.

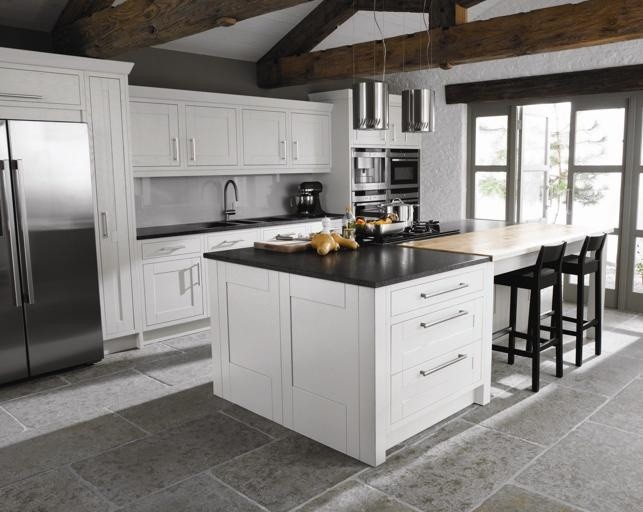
290;182;324;216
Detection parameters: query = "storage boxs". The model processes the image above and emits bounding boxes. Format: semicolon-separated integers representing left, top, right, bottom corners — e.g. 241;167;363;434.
240;95;332;173
129;85;240;176
262;218;347;243
138;228;262;333
374;256;493;469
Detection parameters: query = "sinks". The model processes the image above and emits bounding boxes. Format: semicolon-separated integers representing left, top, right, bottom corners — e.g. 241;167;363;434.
245;217;295;222
204;221;259;229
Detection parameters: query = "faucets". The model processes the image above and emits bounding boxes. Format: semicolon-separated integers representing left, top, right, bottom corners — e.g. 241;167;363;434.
223;179;239;221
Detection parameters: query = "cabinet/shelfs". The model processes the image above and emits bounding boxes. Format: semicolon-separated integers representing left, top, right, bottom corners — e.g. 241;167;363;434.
350;90;422;150
86;58;144;355
0;47;84;121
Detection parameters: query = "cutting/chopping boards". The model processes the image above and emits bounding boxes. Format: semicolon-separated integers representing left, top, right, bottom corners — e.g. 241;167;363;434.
254;238;313;253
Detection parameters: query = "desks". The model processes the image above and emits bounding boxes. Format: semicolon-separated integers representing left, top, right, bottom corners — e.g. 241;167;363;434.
393;222;607;261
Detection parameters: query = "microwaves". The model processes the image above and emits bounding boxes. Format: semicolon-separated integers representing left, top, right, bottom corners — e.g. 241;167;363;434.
353;151;389;204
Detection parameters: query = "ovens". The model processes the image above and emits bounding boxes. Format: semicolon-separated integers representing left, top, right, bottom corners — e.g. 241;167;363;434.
388;147;421;201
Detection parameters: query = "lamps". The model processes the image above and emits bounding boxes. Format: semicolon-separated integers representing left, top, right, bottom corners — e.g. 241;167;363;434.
402;1;436;132
353;1;389;130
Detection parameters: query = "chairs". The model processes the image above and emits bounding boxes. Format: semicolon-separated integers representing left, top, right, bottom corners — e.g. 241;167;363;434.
493;242;568;393
527;234;608;367
